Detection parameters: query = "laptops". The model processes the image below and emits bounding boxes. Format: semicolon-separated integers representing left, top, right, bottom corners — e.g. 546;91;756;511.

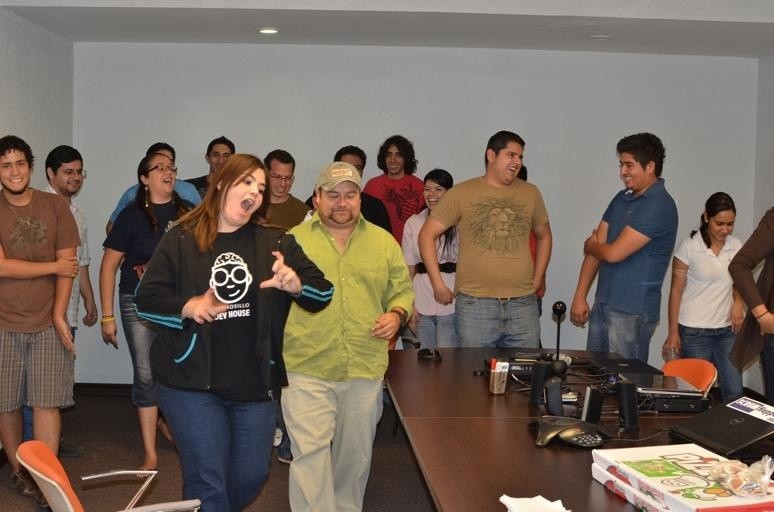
588;358;664;375
617;372;703;399
669;395;774;462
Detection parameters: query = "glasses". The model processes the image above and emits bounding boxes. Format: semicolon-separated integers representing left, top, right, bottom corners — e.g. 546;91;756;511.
145;163;177;175
55;169;86;178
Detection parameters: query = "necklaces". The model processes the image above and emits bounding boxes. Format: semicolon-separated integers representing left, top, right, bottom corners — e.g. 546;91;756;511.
10;205;32;226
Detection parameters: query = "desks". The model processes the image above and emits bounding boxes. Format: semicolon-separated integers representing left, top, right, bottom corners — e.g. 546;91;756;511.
404;417;774;512
385;347;712;418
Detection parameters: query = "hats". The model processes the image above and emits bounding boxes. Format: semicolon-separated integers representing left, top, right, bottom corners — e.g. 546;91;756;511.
315;161;362;192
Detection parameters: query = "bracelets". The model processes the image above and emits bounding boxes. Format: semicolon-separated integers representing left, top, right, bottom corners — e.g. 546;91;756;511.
753;308;770;324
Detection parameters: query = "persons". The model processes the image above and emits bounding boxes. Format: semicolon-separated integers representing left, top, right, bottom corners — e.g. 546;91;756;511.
729;206;774;406
1;135;75;509
21;145;98;457
664;192;747;401
570;134;679;361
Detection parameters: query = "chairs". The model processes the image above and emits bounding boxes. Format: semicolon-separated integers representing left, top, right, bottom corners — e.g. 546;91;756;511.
15;441;201;512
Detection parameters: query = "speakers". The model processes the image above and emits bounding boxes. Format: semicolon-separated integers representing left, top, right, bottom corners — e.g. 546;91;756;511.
531;361;554;405
617;383;641;432
580;385;603;423
543;375;563;417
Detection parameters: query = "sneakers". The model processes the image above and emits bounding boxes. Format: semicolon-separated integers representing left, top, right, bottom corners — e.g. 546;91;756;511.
272;427;283;447
9;473;50;508
277;454;292;464
58;438;86;458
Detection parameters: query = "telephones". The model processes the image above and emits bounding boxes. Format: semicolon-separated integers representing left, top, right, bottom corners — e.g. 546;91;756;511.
528;416;612;448
